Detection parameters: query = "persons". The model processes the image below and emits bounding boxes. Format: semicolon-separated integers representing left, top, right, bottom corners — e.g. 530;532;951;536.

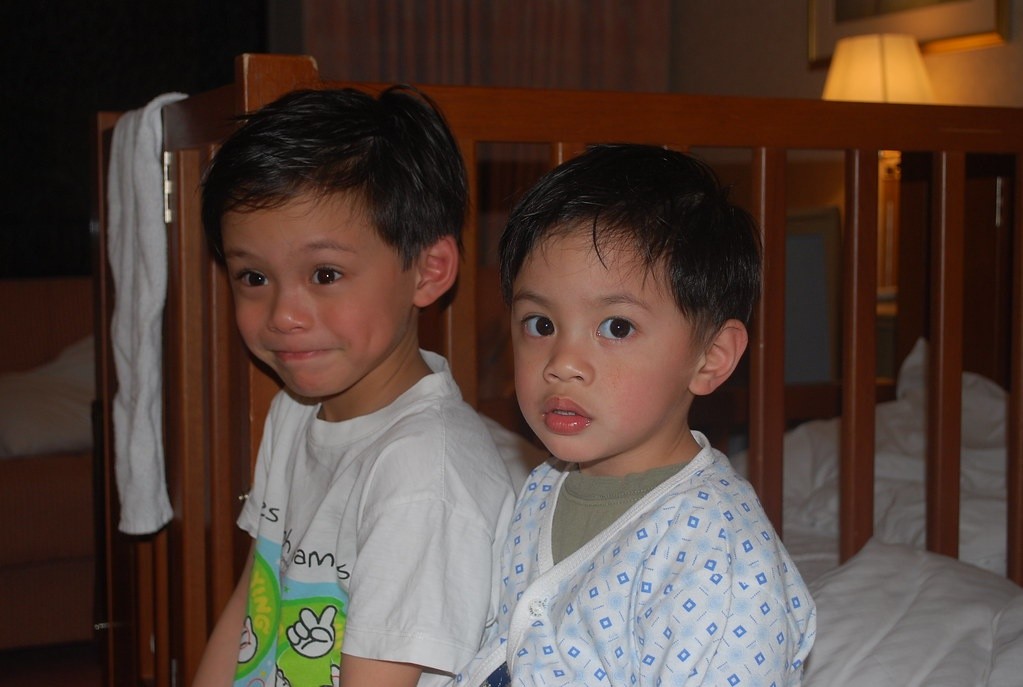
190;89;519;687
438;141;818;687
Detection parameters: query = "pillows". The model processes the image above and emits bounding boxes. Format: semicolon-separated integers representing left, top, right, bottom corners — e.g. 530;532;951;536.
0;334;96;460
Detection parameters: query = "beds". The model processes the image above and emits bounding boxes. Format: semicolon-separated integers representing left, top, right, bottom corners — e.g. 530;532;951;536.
97;53;1023;687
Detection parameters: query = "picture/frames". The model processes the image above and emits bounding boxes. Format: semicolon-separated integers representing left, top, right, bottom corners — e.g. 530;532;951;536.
809;0;1011;68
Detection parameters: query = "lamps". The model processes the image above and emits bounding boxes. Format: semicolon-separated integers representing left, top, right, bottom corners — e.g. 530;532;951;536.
819;33;944;183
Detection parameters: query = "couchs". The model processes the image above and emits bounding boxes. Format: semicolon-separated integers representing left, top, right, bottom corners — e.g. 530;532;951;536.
0;279;98;650
